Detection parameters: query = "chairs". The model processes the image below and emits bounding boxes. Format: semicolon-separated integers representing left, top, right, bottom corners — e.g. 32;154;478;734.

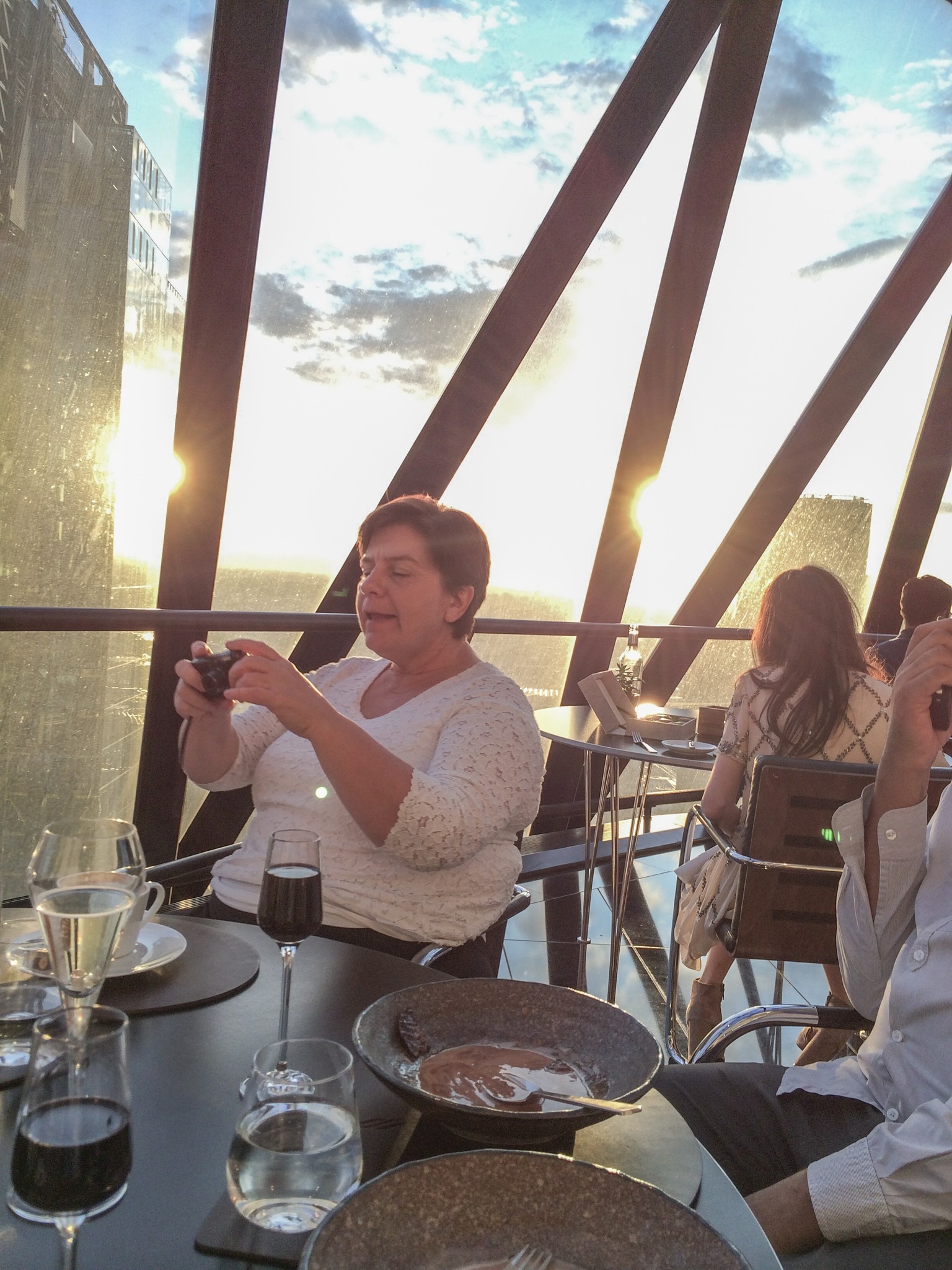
34;754;952;1061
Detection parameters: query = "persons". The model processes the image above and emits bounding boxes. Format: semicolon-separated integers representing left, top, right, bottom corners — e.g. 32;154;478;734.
655;618;952;1270
871;574;952;687
675;565;894;1062
173;495;544;977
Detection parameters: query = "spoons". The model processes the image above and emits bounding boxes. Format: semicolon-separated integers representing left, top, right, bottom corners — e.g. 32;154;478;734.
482;1072;642;1115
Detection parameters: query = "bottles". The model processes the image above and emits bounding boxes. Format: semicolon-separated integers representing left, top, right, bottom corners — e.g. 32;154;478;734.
617;623;643;709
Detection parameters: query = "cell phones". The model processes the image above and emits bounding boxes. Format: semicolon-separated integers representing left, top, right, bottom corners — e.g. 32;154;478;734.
930;685;952;732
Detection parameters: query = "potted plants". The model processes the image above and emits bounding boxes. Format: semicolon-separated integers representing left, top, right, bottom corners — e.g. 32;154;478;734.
614;662;645;709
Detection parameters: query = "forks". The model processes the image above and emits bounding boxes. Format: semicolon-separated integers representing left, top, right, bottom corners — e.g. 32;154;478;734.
632;731;658;753
503;1243;554;1269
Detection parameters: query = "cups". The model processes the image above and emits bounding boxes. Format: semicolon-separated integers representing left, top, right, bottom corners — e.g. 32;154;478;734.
57;869;165;957
226;1037;364;1234
0;918;62;1067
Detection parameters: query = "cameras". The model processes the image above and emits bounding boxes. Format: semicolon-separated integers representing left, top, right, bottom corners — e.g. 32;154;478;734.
193;651;248;700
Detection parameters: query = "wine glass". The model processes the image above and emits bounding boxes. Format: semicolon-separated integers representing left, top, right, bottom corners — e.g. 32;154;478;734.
256;829;323;1102
24;818;148;1096
7;1003;132;1270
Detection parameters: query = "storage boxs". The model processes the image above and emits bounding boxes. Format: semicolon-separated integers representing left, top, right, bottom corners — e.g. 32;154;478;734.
697;706;729;737
577;671;699;742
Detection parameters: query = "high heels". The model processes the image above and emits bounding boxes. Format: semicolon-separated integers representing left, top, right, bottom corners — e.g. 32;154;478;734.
791;994;857;1067
686;978;726;1062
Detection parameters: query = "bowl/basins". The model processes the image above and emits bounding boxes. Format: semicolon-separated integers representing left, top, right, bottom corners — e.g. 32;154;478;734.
351;977;664;1146
297;1149;751;1269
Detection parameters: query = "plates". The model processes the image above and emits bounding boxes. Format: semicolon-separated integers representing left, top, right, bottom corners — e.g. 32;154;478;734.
7;921;188;979
661;739;716;755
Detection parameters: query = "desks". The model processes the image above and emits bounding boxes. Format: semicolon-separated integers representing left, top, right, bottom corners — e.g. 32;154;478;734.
532;705;716;1003
0;908;783;1270
